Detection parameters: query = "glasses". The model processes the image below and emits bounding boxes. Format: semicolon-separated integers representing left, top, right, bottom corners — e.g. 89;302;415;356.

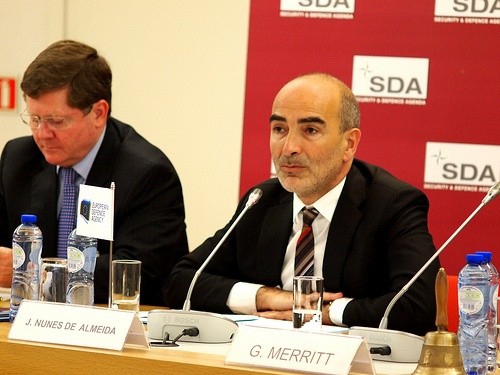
19;108;85;129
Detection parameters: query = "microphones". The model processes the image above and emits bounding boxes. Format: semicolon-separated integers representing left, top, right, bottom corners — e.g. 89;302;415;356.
147;188;263;343
348;181;500;364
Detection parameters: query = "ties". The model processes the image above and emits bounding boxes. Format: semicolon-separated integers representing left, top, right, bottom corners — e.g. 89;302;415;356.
57;167;80;261
293;207;320;295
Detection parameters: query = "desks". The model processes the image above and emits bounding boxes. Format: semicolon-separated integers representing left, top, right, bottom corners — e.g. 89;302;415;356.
1;300;350;375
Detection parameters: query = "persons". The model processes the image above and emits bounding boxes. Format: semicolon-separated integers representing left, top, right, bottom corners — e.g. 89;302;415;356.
164;72;442;335
0;40;189;310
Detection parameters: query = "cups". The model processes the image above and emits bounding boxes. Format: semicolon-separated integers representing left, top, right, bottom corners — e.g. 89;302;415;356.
292;276;324;334
39;257;67;302
110;259;142;313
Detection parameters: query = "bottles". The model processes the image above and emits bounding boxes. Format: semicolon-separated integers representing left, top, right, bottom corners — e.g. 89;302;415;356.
475;252;499;374
65;217;97;307
9;214;43;326
457;253;489;375
